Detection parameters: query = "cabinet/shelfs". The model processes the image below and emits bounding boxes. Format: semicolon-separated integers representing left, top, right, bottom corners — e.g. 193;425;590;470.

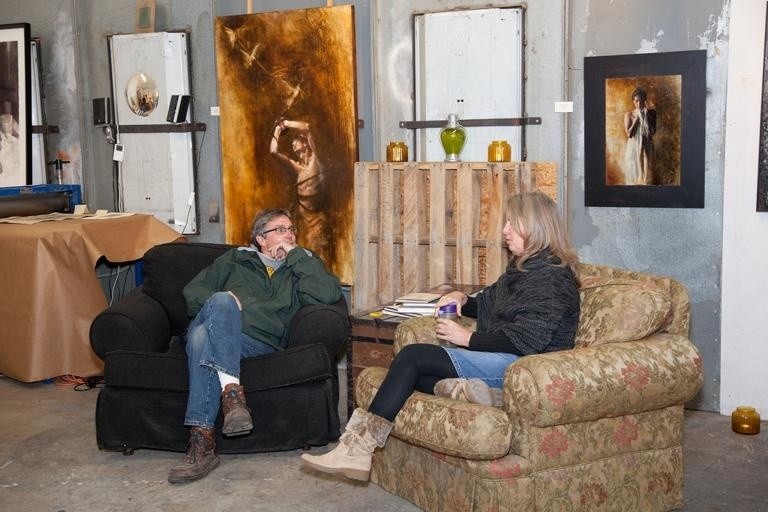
346;282;486;421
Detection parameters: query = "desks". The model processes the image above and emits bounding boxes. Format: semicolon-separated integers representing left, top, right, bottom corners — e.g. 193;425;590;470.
2;212;191;384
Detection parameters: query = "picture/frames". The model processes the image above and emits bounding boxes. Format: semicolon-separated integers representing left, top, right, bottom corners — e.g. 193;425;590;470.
583;49;707;209
135;1;154;32
0;22;33;189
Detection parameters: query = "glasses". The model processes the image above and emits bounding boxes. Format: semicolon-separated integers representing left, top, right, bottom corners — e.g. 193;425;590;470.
263;225;298;235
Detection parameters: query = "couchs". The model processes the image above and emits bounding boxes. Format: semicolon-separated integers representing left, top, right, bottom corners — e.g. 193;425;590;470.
91;243;352;455
356;262;704;511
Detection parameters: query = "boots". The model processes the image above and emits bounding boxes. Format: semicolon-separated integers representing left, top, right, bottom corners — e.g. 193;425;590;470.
218;383;254;438
299;406;395;483
166;428;220;485
433;375;506;408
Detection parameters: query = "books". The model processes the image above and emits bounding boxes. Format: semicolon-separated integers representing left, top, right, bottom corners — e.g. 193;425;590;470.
382;293;443;319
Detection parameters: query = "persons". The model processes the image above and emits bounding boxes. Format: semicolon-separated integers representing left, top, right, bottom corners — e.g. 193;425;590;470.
269;117;332;271
300;192;581;483
168;209;344;485
624;87;657;187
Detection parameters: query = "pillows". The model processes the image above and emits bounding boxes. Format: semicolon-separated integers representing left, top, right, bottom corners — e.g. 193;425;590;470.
574;280;670;350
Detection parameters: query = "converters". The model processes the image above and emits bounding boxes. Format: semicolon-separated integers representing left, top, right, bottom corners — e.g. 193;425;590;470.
87;378;97;388
188;191;195;206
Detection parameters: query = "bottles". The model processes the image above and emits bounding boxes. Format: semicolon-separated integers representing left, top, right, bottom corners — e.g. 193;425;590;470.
440;305;459;348
731;405;760;436
487;140;511;162
386;140;409;162
440;113;469;162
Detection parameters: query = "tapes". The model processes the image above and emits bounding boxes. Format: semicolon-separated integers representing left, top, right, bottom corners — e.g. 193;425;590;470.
370;312;383;317
